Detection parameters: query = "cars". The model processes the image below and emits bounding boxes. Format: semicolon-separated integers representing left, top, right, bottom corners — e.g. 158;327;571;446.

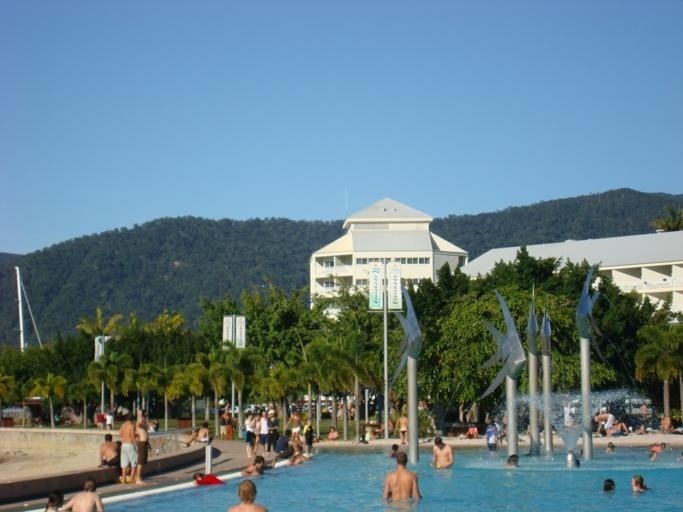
226;399;377;420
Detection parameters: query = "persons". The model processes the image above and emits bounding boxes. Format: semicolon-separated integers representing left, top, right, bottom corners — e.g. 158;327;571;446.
592;402;673;461
430;437;454;470
327;426;339;441
382;452;422;501
383;501;422;512
221;401;315;476
226;480;268;512
58;478;105;512
507;455;520;468
195;424;212;442
364;415;408;445
602;479;614;490
44;491;72;512
463;420;503;450
630;475;647;492
95;407;159;485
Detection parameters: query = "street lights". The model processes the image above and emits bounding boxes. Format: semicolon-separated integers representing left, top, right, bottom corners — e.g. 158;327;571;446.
21;395;45;426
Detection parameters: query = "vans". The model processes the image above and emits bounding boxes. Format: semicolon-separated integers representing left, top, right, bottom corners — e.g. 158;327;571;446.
599;398;652;416
